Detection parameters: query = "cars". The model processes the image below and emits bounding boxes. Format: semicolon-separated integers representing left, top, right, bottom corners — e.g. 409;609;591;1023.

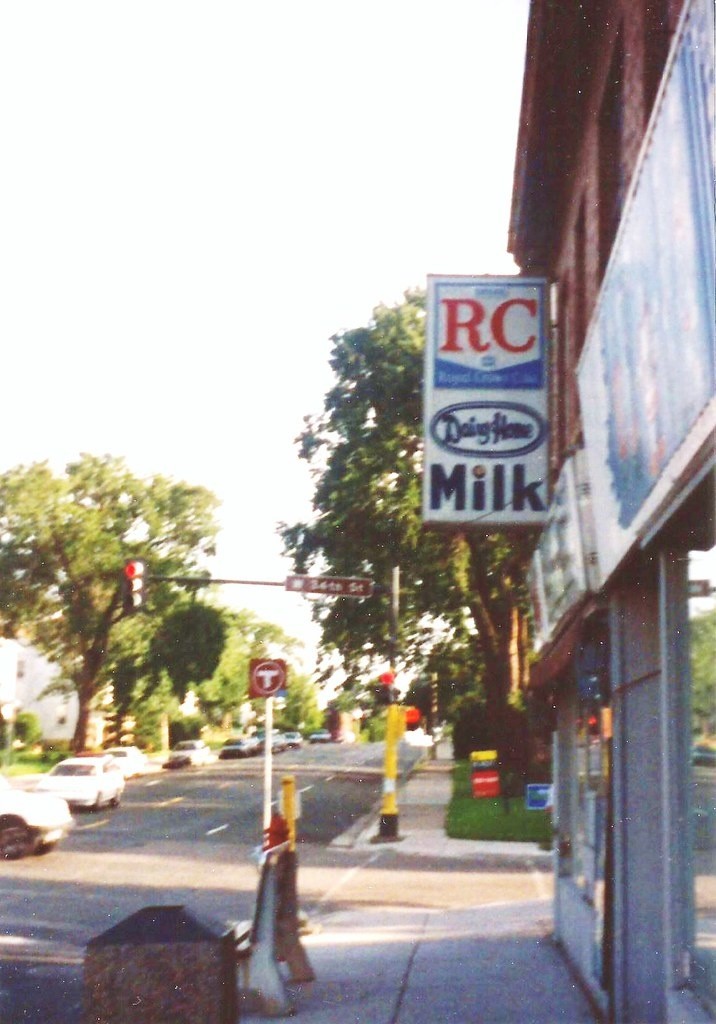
103;746;149;780
0;773;75;859
45;754;127;811
220;727;303;758
309;728;332;743
168;740;212;766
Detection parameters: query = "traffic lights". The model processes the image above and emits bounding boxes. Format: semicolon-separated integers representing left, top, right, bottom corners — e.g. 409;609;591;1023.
122;556;145;613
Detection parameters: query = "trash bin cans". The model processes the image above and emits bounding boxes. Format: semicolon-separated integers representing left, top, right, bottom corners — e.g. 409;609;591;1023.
85;906;240;1024
